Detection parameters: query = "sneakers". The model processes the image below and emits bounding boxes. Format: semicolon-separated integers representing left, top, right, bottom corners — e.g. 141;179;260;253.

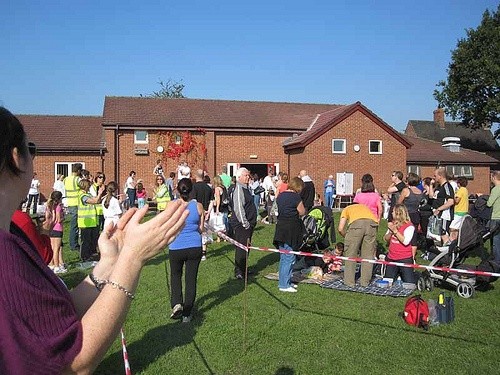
235;270;245;280
291;283;298;288
279;286;298;292
169;303;183;319
182;313;193;322
48;260;67;273
247;266;254;274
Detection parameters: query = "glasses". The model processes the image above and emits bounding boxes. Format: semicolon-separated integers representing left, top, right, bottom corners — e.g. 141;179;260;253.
98;176;104;179
157;178;163;180
22;142;36;159
392;175;395;177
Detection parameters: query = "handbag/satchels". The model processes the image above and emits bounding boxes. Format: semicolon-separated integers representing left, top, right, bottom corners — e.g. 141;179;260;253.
426;214;443;241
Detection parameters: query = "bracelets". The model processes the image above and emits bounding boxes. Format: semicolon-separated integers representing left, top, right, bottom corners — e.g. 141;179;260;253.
104;279;135;300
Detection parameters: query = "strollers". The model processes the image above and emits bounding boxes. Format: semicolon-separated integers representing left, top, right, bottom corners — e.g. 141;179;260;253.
417;214;499;298
290;206;334;254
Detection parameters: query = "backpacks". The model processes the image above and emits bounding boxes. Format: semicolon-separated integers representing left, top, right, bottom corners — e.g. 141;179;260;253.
403;294;430;331
435;292;454;323
42;203;58;231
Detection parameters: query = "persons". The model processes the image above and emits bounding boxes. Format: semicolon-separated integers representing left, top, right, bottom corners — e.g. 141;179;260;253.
248;167;344;275
485;170;500;267
165;178;204;323
0;106;190;375
383;204;424;285
11;196;59;266
352;167;469;265
26;159;237;275
272;177;305;292
230;166;257;281
338;202;379;288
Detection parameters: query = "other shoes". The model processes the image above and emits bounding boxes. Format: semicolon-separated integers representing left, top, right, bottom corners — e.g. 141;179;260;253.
70;245;99;268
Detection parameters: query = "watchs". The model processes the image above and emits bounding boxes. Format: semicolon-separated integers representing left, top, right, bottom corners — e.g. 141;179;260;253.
89;271;107;292
